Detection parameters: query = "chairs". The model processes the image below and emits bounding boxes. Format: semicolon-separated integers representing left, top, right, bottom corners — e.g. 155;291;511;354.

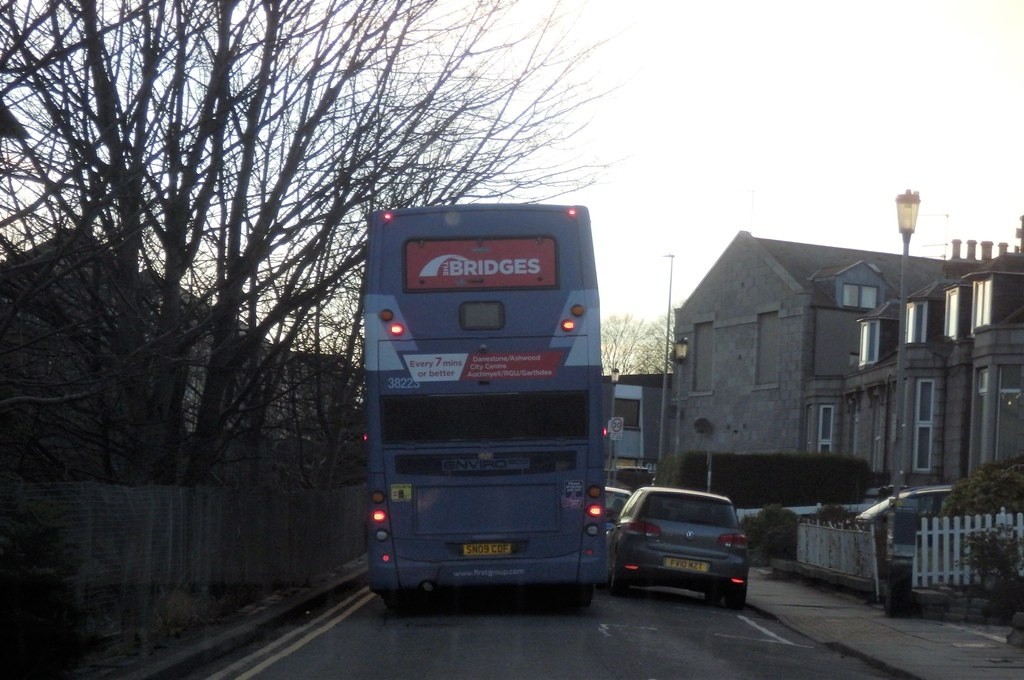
653;509;678;523
684;506;713;524
612;499;624;512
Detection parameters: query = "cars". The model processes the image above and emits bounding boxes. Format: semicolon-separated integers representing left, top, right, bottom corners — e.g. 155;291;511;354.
603;485;633;533
605;487;750;611
855;484;952;533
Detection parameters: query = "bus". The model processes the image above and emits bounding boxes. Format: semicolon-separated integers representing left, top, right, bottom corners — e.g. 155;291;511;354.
360;204;611;613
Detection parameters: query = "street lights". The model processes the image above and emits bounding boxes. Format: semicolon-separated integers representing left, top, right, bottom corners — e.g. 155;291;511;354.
895;190;921;506
672;337;690;454
606;366;619;483
657;254;675;463
